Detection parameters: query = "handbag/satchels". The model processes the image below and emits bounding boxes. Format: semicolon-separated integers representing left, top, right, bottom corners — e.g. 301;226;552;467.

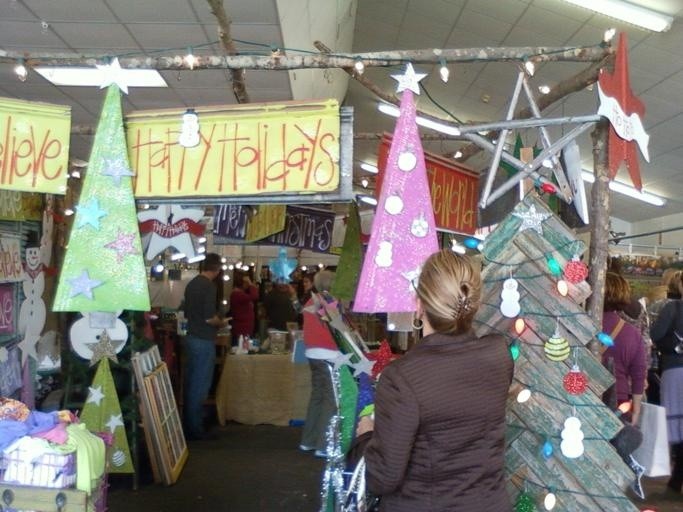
631;392;672;477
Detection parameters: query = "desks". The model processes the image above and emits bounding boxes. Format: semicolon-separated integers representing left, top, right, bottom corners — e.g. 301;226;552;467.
220;349;312;426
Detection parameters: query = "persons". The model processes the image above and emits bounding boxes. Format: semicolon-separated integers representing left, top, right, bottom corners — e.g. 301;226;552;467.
183;253;342;457
601;270;683;489
352;248;514;512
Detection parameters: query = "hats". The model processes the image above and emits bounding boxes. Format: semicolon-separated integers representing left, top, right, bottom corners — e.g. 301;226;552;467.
203;254;225;265
25;229;40;248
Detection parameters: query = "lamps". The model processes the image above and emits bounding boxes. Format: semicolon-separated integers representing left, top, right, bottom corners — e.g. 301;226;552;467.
542;158;667;207
563;0;674;34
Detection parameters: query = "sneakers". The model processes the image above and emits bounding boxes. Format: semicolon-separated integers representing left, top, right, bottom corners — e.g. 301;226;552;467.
298;444;327;457
185;432;209;439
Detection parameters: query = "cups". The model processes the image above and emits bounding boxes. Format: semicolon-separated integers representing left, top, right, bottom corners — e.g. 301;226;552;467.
270;331;286;355
